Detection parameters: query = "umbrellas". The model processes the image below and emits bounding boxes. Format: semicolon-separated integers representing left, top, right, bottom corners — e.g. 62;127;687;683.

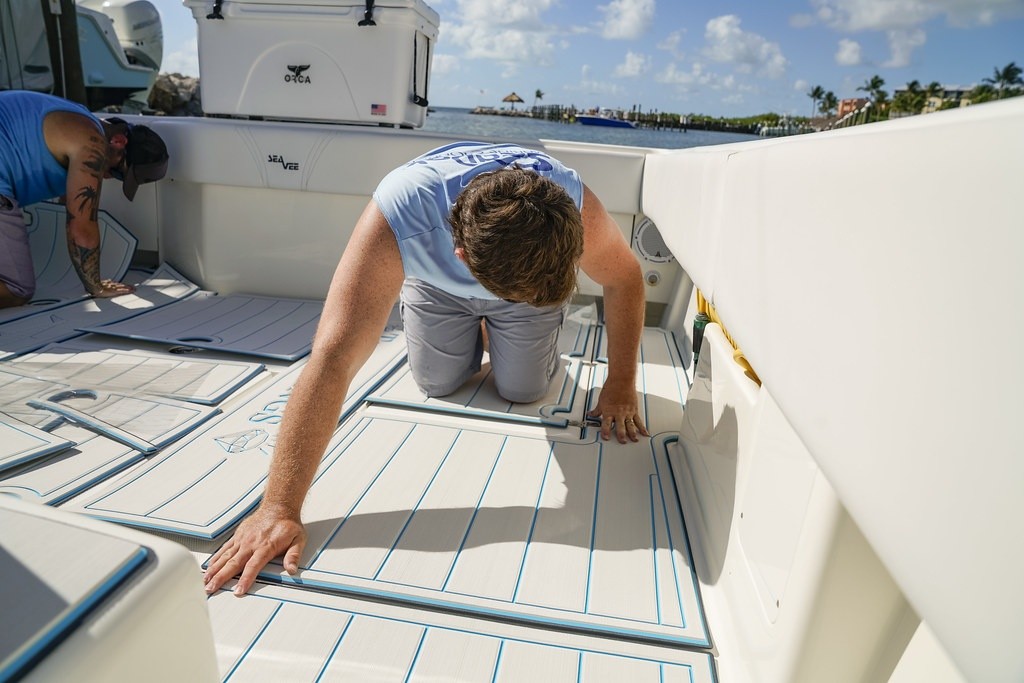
503;92;525;111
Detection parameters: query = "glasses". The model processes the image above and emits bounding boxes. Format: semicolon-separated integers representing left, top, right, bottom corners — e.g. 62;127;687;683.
109;150;126;180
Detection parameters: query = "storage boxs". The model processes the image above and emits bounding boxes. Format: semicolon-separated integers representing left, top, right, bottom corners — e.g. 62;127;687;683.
182;0;440;131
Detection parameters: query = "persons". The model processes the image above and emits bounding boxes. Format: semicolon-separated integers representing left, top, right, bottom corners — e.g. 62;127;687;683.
202;140;651;598
0;91;170;308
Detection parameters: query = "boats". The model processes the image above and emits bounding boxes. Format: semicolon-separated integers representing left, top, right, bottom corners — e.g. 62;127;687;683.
575;108;640;129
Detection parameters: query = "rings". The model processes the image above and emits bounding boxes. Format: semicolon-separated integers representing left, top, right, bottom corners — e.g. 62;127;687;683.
625;419;634;422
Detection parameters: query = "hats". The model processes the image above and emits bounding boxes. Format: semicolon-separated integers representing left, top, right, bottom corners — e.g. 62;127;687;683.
123;124;170;202
